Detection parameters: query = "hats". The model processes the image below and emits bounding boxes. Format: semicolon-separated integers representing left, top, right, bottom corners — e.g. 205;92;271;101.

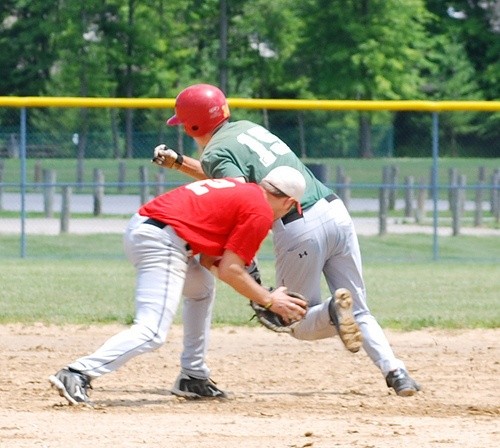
265;165;306;214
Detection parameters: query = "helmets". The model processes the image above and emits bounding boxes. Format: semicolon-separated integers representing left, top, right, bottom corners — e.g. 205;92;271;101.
167;83;231;137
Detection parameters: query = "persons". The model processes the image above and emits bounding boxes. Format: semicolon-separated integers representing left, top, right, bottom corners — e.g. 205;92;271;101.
152;83;420;395
49;165;308;406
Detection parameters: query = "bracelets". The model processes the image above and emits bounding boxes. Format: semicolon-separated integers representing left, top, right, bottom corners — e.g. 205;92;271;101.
173;153;184;169
266;293;274;308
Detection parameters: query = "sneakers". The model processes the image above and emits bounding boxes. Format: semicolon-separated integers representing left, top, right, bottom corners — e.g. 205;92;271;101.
171;372;236;401
48;367;94;409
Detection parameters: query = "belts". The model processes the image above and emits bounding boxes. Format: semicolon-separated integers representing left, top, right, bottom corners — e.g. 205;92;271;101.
144;217;192;251
281;193;338;225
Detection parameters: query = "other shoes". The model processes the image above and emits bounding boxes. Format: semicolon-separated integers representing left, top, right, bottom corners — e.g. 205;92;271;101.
329;287;363;353
391;367;421;396
